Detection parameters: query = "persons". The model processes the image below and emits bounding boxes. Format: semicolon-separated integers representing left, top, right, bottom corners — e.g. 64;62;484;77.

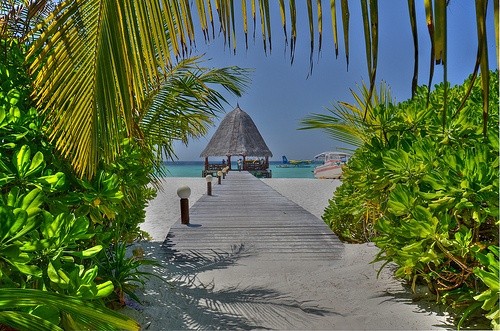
261;159;264;165
238;159;242;171
222;159;226;167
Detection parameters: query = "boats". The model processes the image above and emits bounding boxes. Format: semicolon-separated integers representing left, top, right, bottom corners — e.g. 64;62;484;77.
314;151;352;180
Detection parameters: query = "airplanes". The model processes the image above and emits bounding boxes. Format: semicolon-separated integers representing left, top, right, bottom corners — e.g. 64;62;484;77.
283;155;312;168
